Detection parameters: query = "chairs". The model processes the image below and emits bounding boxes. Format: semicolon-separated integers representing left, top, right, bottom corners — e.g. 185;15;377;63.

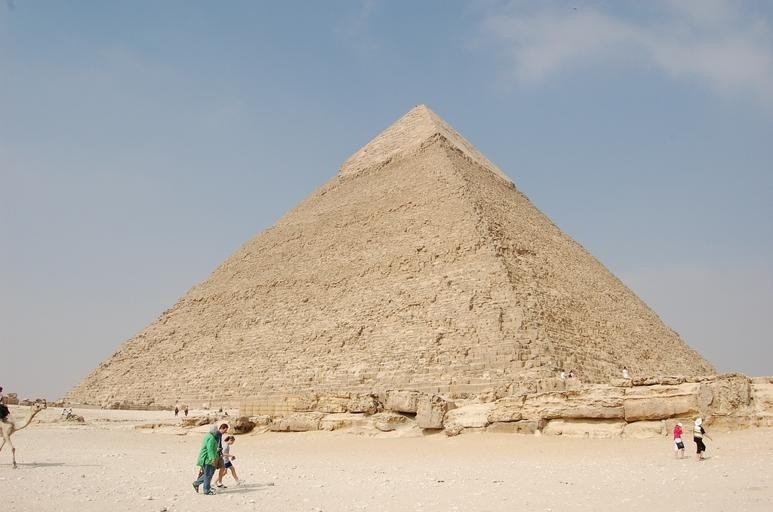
193;479;244;494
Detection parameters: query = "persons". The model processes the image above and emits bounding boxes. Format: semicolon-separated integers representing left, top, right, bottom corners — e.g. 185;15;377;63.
692;417;711;461
215;434;244;488
620;365;630;386
0;387;9;421
672;422;685;460
214;422;226;489
190;428;220;495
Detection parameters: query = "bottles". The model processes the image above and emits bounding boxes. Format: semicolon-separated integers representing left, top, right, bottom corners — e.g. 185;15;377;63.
695;418;702;426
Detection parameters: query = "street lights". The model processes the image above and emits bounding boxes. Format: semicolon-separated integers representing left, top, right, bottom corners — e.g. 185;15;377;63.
218;458;224;468
